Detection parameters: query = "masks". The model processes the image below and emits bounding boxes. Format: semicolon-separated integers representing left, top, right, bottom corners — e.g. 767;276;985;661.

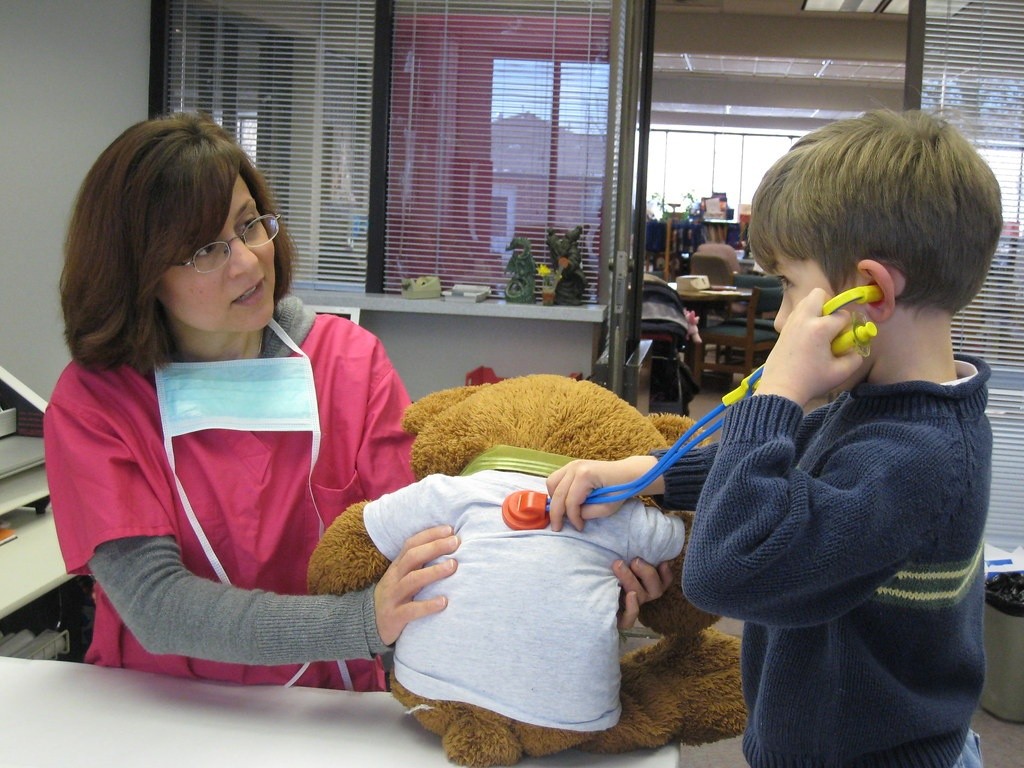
155;320;319;436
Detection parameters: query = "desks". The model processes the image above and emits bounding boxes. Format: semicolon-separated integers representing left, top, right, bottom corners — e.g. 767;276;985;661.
667;282;753;388
0;657;681;768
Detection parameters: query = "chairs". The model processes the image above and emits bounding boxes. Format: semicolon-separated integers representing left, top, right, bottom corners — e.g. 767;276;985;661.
641;214;784;389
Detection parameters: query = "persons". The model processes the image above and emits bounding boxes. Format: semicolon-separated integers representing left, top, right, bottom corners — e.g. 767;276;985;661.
544;107;1004;768
40;109;460;695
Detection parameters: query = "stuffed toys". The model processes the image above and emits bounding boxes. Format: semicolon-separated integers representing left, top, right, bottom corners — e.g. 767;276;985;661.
304;372;748;767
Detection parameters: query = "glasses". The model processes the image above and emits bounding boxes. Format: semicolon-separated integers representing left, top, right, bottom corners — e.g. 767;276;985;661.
177;209;281;273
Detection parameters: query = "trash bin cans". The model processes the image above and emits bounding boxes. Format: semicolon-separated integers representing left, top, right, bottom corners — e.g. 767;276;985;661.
980;569;1024;731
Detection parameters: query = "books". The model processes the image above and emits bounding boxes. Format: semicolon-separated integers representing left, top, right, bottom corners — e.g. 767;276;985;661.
672;223;741;253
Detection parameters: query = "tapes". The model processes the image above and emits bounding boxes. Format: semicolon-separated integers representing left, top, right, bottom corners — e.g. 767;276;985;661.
416;276;429;288
